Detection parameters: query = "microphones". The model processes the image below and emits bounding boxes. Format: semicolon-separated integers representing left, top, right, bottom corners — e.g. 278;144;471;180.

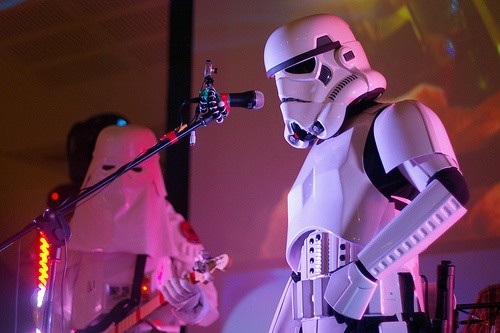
184;89;265;110
431;260;455;333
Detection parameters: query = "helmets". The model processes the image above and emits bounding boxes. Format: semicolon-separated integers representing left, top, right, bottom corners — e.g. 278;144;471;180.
260;13;390;149
74;122;170;214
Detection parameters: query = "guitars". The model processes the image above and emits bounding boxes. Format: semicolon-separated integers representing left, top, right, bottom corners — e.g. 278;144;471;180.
70;248;230;333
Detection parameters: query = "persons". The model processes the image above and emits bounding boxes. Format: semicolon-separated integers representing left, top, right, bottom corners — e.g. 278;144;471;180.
33;123;219;332
263;13;472;333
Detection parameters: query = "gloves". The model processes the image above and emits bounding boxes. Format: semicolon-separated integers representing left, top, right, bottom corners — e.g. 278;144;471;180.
158;278;204;317
322;265;378;329
